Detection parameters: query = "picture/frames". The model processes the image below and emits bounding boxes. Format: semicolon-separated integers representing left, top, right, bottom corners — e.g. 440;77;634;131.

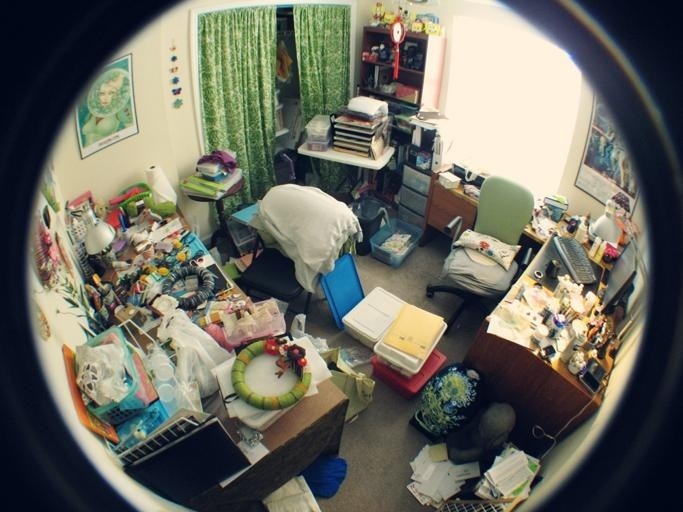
75;53;140;160
573;89;640;220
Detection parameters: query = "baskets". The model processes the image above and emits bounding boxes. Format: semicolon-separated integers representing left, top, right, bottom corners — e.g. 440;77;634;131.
73;327;150;426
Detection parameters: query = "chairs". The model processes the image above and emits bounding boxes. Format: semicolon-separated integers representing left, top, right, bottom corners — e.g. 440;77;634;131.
241;230;322;317
426;175;534;335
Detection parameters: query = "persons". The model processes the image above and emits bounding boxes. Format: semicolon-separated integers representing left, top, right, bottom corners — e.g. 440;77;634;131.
80;67;131;145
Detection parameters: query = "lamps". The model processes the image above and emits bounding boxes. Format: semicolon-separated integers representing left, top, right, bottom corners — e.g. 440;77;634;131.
590;200;647;344
71;206;117;256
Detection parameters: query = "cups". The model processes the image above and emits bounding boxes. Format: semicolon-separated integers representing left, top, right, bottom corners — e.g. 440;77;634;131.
545;258;561;279
98;284;114;307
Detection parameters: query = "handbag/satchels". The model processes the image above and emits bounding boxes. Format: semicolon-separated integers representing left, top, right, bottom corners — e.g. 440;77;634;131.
314;345;375;424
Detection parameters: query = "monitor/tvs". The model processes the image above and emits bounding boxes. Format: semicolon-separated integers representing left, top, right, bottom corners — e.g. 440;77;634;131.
600;240;636;312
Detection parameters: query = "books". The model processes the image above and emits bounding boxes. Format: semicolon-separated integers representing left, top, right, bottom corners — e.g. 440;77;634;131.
375;232;412;264
198;261;232;298
181;171;242;201
383;303;445;361
472;447;541;509
332;110;375;158
124;415;251;505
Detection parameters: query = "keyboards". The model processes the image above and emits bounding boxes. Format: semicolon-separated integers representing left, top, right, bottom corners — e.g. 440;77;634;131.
553;236;598;284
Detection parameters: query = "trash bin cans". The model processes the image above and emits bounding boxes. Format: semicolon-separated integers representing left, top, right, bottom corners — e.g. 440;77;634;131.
353;196;385;256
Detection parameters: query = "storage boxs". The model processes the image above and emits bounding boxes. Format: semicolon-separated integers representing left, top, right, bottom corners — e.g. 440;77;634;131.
350;163;432;270
275;104;284;131
305;114;333;152
342;287;447;401
274;89;281;108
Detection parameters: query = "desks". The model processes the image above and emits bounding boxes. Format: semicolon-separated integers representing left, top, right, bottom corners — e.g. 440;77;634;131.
462;208;619;458
189;176;245;258
297;140;395;195
120;204;350;512
274;128;289;155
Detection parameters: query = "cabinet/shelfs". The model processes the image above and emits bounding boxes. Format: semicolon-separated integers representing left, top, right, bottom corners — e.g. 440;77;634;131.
418;178;480;247
357;24;449;210
